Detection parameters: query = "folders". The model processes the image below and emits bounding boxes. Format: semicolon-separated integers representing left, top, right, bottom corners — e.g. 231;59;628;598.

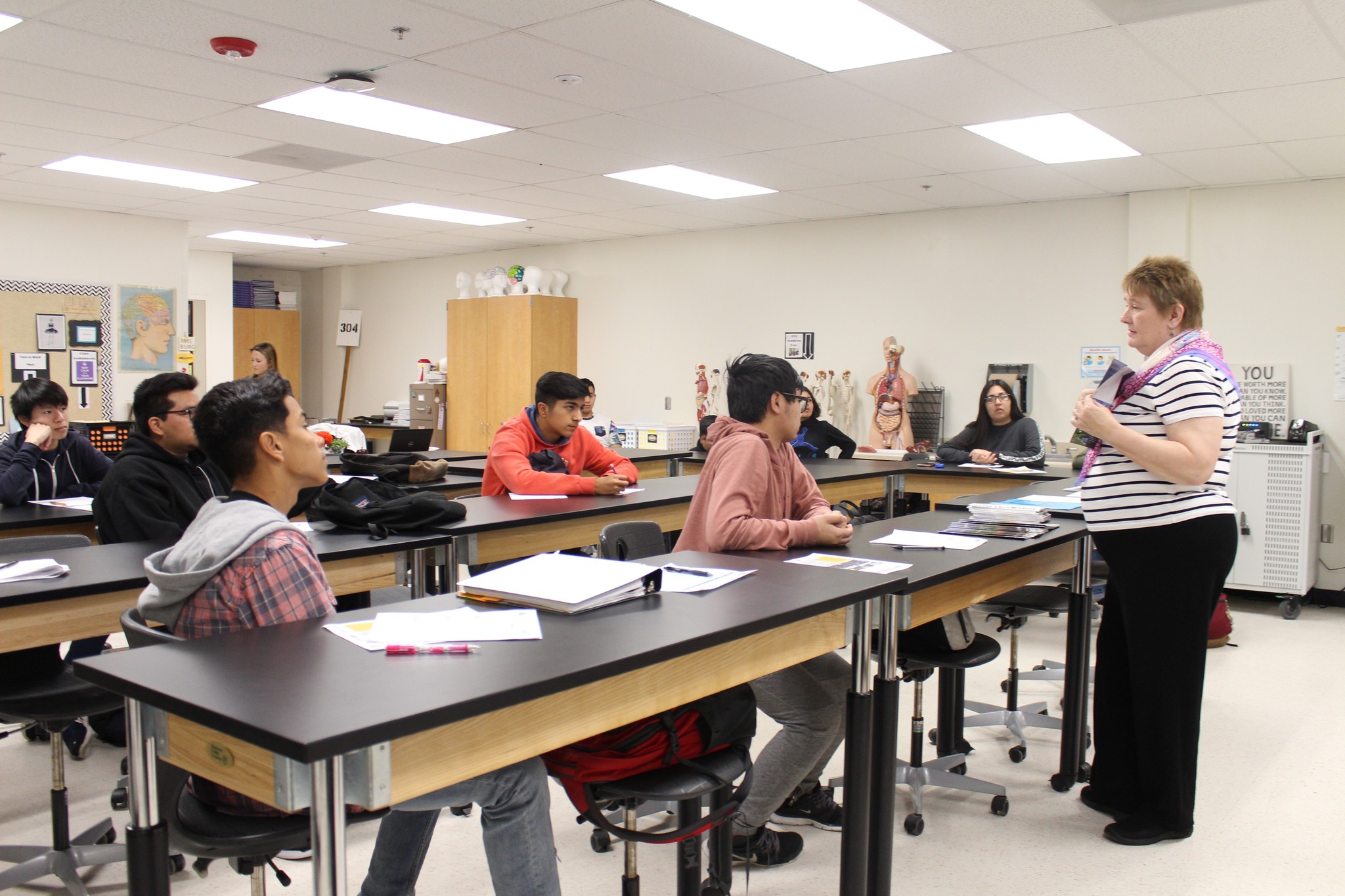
455;552;662;615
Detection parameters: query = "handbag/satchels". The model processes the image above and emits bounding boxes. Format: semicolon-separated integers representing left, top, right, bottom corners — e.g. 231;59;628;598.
1206;594;1233;640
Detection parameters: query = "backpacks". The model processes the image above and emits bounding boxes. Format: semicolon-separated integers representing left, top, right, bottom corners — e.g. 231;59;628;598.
340;449;448;482
839;499;977;661
529;449;570;475
306;476;468;541
538;677;757;844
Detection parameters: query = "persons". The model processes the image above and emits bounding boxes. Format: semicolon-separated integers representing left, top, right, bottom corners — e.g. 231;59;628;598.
468;371;639;577
936;379;1045;468
578;378;622;447
688;415;717;452
0;377;116;760
94;372;232;551
672;353;853;871
1071;257;1238;846
250;342;293;399
137;372;561;896
1073;448;1090;469
788;386;856;459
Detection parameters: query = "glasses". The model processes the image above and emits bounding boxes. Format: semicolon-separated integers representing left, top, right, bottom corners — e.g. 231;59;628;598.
159;407;196;420
983;393;1011;404
782;391;809;414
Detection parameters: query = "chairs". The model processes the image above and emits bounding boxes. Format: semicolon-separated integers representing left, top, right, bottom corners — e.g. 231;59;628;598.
0;536;185;896
600;521;666;561
118;613;391;896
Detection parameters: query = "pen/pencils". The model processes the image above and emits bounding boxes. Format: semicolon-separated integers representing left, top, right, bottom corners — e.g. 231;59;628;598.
973;463;995;465
891;546;945;550
48;501;68;505
0;561;18;569
386;645;484;655
610;464;616;474
663;566;713;577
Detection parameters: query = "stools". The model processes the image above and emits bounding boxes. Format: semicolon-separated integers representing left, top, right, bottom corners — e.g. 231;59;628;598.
593;745;756;896
929;585;1092;762
822;618;1008;835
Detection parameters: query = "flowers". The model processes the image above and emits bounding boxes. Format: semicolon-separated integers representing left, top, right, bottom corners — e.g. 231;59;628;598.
318;431;334;445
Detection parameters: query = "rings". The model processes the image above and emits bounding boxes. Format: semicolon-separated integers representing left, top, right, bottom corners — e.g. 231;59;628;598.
1076;413;1078;416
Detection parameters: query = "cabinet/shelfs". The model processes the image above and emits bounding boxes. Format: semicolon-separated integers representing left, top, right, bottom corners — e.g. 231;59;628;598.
232;308;300;408
446;295;578;453
410;384;444;449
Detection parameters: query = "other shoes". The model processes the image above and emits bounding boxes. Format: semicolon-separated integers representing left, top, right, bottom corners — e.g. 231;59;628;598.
1104;822;1193;846
1080;785;1121;816
278;842;313;859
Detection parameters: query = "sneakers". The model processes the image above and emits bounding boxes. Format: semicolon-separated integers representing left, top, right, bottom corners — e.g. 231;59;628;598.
62;719;92;758
22;722;47;743
702;825;804;866
772;782;844;831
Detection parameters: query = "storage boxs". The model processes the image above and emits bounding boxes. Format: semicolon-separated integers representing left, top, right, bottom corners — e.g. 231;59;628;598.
614;423;696;451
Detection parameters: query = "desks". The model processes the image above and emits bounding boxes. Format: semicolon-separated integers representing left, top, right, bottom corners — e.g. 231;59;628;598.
0;422;1099;896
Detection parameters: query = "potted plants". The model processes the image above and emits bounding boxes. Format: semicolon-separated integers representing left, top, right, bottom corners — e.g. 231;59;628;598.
332;439;348;453
